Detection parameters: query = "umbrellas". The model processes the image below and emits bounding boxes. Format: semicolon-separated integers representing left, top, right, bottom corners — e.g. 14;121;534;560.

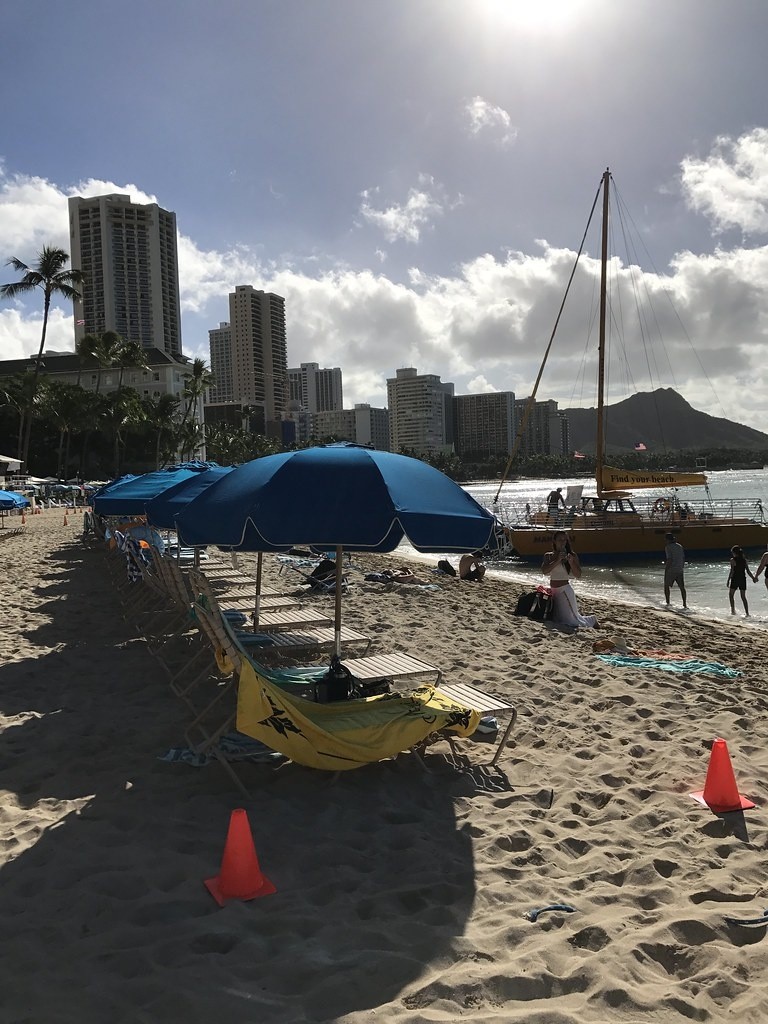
170;440;497;662
51;459;221;519
141;461;262;633
0;490;31;529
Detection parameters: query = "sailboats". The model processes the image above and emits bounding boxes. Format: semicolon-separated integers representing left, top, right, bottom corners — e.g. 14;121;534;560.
490;166;768;562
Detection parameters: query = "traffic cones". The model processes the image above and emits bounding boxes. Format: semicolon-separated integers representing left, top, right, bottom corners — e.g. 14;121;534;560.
688;737;756;815
64;508;69;515
203;806;277;905
35;508;40;514
73;507;77;514
88;507;91;512
80;507;83;513
62;515;69;526
21;513;25;524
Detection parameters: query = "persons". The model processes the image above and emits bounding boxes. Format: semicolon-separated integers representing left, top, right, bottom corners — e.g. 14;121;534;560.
726;545;757;617
544;487;566;527
459;550;485;582
663;531;690;608
542;528;601;629
753;550;768;588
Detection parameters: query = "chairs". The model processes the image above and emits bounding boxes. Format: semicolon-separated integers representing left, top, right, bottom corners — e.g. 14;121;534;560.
27;499;517;801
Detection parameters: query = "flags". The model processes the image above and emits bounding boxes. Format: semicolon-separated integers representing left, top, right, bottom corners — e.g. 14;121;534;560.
634;443;647;450
